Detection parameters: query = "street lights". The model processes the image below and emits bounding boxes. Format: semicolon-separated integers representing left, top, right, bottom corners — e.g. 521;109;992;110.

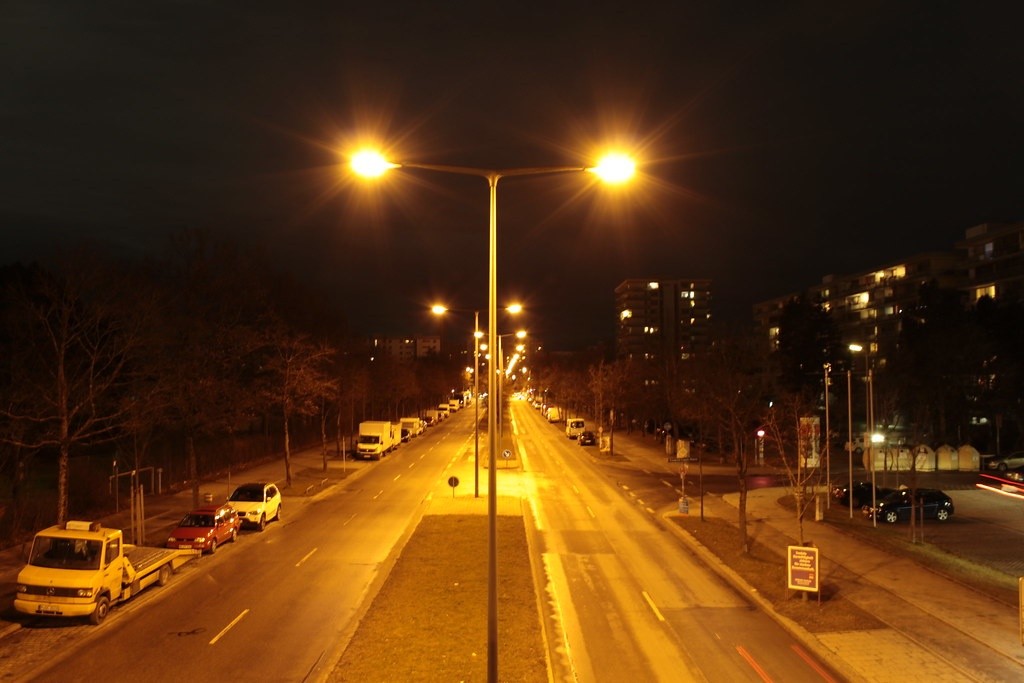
430;301;523;497
849;343;871;480
473;328;527;439
347;145;638;683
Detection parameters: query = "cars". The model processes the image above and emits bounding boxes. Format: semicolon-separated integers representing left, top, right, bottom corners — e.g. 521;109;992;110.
166;503;240;555
223;482;282;531
401;428;411;442
576;431;596;446
984;449;1024;472
832;480;898;508
528;397;562;422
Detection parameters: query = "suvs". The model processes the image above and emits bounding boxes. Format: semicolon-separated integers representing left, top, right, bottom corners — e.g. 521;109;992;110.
861;486;955;524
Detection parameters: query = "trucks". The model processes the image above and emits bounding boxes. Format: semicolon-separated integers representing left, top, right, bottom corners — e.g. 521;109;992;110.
13;520;202;626
400;391;471;438
354;420;402;460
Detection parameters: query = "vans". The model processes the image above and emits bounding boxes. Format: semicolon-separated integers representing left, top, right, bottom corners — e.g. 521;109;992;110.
566;418;586;439
